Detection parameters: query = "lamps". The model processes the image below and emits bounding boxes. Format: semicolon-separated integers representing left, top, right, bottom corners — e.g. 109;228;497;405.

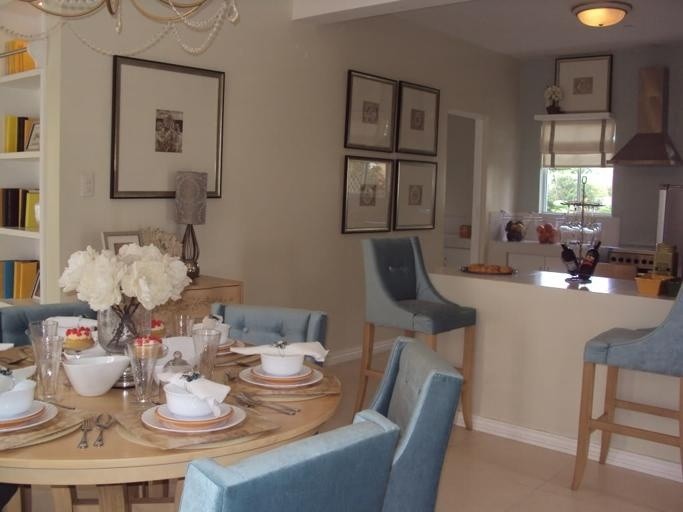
172;169;208;282
571;1;631;29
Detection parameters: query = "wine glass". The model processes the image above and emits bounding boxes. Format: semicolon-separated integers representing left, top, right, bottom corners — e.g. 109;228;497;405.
557;200;602;243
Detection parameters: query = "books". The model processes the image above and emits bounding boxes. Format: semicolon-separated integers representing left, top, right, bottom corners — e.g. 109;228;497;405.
0;39;42;300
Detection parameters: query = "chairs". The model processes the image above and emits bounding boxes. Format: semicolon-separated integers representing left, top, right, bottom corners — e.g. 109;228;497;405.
177;407;391;511
203;300;322;363
0;303;96;347
570;281;683;494
354;238;476;435
367;333;466;511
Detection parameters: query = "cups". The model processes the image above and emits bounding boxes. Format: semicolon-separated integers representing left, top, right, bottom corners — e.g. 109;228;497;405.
190;329;222;379
533;212;557;244
171;313;191;337
500;215;529;242
459;224;470;238
27;319;59;339
125;339;159;402
30;334;64;400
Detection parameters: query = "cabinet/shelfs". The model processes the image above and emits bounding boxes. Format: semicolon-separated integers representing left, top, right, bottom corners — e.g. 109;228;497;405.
153;271;242;321
506;254;567;273
0;64;45;244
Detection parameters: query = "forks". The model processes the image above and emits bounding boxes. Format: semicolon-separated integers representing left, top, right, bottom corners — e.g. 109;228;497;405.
0;355;28;365
233;392;301;415
75;413;93;448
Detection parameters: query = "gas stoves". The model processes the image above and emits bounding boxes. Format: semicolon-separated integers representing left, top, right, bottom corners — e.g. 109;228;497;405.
609;246;655;269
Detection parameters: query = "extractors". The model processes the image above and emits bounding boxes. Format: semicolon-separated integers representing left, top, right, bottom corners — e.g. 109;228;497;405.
606;66;683;167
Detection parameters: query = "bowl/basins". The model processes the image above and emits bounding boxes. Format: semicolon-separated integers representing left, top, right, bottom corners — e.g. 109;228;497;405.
161;382;215;416
0;378;36;417
152;336;197;380
259;352;306;375
190;323;231;344
43;315;97;340
634;273;681;296
62;353;128;399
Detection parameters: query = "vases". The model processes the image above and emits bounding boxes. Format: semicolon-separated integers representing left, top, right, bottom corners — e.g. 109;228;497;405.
546;101;559;113
98;302;157;388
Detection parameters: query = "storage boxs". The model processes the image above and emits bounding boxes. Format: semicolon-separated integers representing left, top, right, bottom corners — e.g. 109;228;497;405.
633;273;675;296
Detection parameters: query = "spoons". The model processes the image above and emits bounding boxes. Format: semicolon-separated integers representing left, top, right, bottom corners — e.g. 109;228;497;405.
94;411;111;448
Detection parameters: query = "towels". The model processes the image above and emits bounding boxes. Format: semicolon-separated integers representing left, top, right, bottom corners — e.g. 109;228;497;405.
230;342;329;361
157;366;231;414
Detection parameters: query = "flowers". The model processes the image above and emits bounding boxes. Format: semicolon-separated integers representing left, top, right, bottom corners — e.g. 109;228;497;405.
60;247;182;311
543;83;564;103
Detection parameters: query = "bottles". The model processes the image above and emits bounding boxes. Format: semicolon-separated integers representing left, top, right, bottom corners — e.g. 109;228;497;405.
578;239;601;280
560;243;578;277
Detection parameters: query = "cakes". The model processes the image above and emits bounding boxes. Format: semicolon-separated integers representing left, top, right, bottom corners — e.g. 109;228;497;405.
64;327;94;351
151;319;166;338
133;336;163;359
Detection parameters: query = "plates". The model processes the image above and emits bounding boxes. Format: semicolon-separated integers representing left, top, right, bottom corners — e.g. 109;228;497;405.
0;401;58;436
139;401;247;435
238;363;324;390
217;340;246;356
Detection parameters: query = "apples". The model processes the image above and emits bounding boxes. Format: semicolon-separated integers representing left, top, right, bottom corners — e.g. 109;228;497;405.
537;223;555;243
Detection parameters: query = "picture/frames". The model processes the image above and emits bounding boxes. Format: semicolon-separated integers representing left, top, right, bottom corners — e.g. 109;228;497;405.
343;66;398;156
109;53;222;203
98;231;141;254
340;154;392;236
24;122;39;152
394;159;435;233
395;81;440;157
31;271;40;303
550;54;612;112
